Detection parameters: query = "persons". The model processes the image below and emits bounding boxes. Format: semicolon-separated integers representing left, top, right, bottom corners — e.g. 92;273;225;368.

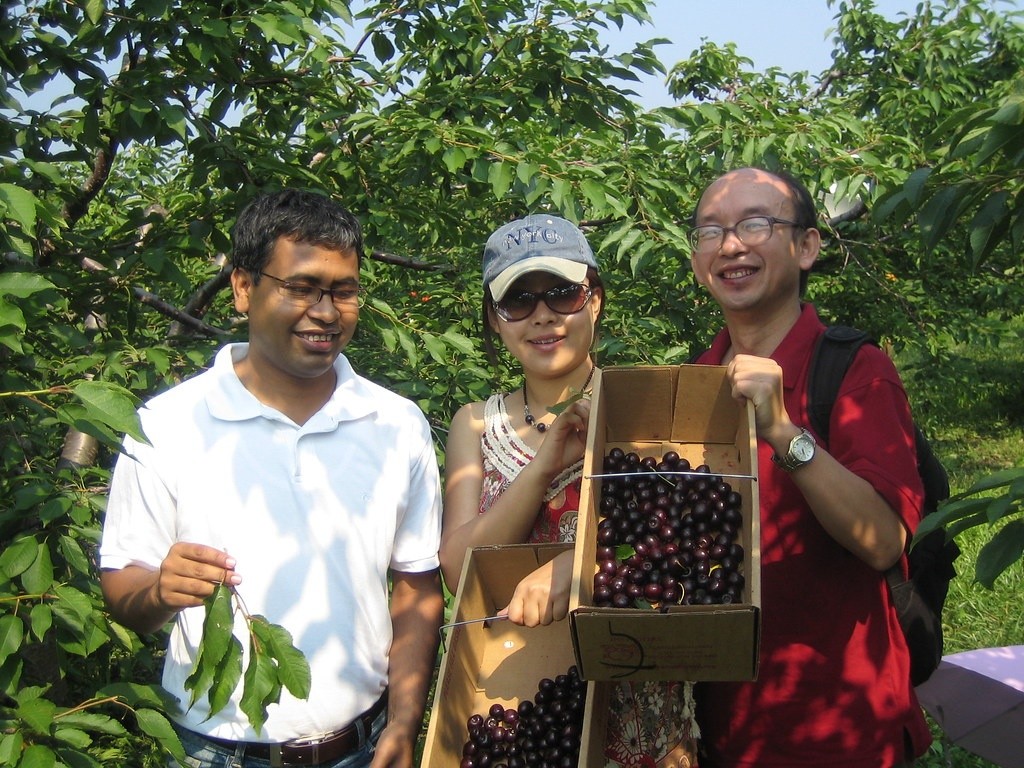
691;166;934;768
441;214;606;627
100;188;444;768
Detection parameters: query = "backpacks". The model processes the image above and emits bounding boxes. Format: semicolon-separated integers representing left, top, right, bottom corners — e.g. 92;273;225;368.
684;324;961;687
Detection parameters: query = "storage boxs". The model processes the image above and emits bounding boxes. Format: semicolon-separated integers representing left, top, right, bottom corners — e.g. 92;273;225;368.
419;542;608;768
565;364;763;684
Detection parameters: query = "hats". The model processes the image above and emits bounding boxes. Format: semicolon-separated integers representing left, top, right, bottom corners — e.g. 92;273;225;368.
482;214;598;302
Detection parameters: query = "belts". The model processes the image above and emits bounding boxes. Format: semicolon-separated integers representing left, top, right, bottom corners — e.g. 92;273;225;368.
191;687;388;765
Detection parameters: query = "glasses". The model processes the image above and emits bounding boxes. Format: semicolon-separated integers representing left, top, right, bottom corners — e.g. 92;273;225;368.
490;283;593;323
257;271;366;310
686;216;807;253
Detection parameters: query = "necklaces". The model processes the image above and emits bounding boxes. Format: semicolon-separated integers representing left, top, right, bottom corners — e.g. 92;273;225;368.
524;364;595;432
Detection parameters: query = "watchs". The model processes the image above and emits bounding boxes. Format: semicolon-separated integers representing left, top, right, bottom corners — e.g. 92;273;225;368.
771;427;816;472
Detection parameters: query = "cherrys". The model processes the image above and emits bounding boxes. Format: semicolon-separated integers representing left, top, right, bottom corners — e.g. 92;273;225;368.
457;664;586;768
592;444;746;608
